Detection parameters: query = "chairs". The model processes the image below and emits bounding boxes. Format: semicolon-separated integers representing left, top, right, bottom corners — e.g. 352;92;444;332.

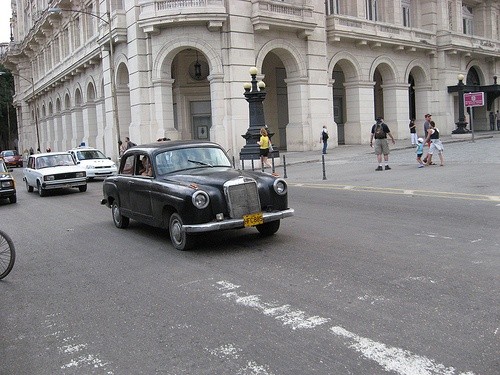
139;157;162;177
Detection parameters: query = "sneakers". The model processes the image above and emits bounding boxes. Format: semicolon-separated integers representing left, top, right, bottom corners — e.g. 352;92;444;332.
375;165;391;171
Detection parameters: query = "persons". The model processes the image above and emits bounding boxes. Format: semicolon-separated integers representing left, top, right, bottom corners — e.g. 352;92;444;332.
322;125;330;154
140;155;153;177
494;110;500;131
415;138;424;168
489;112;495;131
125;136;134;149
423;114;437;165
257;128;273;172
408;119;418;145
118;140;126;156
423;120;445;167
369;116;395;171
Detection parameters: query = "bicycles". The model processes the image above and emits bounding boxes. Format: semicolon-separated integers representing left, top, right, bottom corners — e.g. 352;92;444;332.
0;230;16;279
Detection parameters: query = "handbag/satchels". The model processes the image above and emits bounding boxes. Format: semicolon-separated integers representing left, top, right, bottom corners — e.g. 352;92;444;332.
322;130;329;140
268;140;274;152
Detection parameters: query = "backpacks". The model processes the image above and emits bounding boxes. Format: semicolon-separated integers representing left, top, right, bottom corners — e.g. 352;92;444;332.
374;123;386;139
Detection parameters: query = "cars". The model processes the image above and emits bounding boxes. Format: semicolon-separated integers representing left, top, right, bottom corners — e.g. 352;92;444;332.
69;148;117;179
0;158;17;203
23;152;87;196
1;150;24;169
100;139;295;251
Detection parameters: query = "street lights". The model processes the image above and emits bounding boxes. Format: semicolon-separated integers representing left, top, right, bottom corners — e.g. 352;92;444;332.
451;74;472;133
240;67;280;159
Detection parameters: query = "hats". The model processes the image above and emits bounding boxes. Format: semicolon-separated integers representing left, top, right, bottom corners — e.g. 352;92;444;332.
417;137;424;143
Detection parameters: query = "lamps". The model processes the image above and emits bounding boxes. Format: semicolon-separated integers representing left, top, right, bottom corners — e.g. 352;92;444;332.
193;51;203;80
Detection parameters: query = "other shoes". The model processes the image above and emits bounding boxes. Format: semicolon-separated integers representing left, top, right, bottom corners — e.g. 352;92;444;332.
418;158;444;168
